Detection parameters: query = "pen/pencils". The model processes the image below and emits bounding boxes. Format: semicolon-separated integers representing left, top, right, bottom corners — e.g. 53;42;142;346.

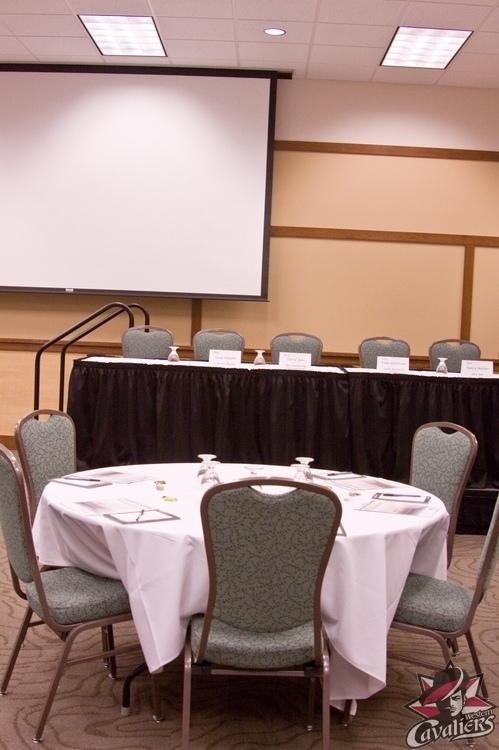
63;476;100;482
136;509;144;521
328;472;353;475
383;494;421;497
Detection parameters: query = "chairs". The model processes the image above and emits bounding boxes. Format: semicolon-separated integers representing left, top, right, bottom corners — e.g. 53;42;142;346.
0;443;164;742
121;325;173;359
193;328;245;361
182;477;342;749
343;493;499;726
410;421;478;647
429;339;481;372
15;409;77;571
270;332;323;366
358;337;410;368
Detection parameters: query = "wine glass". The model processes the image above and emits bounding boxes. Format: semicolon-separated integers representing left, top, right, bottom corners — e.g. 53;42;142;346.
290;463;310;481
168;346;180;361
201;461;220;490
295;457;314;480
436;358;448;374
254;350;266;365
196;454;217;476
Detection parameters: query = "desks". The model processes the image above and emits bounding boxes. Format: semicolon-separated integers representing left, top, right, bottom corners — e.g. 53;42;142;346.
67;355;347;473
30;463;450;717
342;367;499;526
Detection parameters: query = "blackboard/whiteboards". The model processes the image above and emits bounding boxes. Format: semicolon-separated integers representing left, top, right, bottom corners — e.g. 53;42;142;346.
0;62;277;302
